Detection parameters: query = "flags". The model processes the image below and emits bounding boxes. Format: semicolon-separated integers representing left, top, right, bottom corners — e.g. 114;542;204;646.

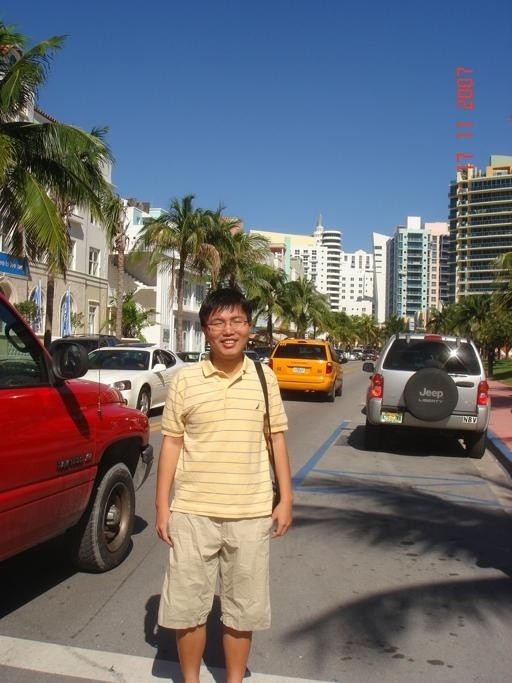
29;285;43;334
59;289;72;338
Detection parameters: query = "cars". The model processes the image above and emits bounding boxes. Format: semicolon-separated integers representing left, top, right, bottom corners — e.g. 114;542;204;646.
69;341;185;419
173;351;209;364
63;334;121;356
247;345;274;365
332;343;382;363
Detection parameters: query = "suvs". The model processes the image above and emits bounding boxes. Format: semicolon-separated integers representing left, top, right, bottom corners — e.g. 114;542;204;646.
0;273;157;574
359;329;492;459
267;337;348;403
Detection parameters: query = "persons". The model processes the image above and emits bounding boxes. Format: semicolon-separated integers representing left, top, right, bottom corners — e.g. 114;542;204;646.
152;284;295;682
